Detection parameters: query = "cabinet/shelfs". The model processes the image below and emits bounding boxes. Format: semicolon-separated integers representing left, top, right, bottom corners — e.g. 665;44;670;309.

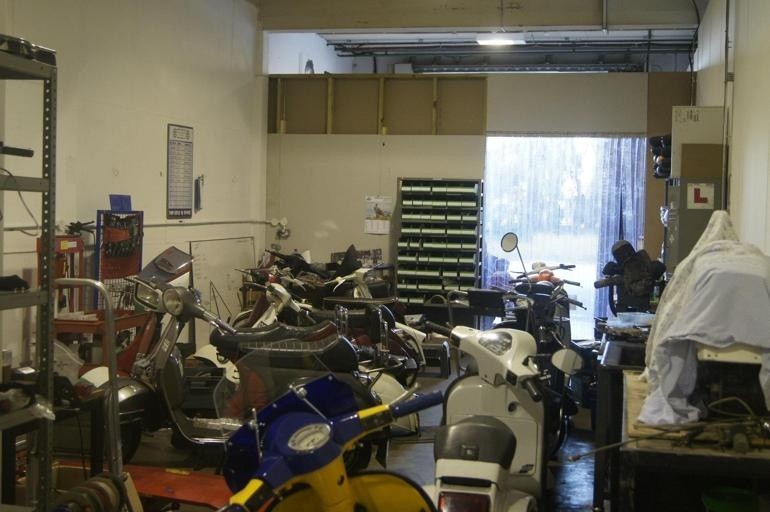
0;51;57;512
392;176;485;299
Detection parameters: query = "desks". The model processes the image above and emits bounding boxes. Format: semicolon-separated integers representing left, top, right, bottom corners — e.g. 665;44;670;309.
56;312;155;378
620;370;770;510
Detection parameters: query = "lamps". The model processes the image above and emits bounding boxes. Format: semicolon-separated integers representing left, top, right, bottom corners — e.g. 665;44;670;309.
476;33;526;47
